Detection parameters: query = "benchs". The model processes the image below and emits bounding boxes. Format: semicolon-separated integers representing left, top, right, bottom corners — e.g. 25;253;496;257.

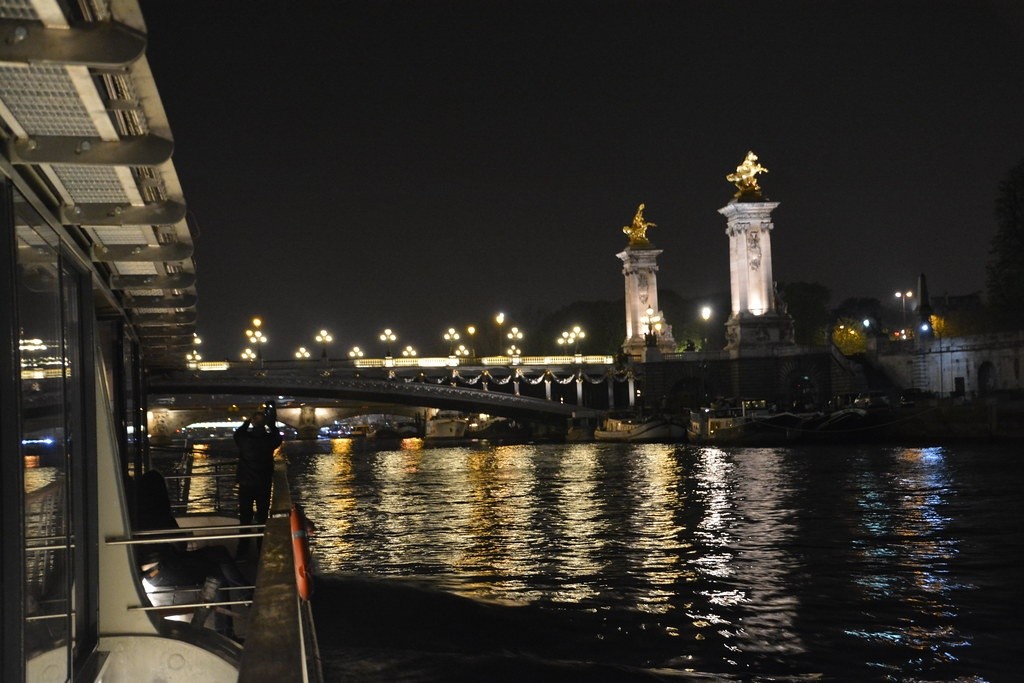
145;515;243;634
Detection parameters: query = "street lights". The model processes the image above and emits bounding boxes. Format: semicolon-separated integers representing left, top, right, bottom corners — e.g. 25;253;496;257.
701;307;711;352
468;326;476;357
496;316;504;355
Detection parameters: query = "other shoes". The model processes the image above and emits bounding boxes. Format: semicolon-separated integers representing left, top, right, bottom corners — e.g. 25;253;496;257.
231;636;245;647
240;597;254;610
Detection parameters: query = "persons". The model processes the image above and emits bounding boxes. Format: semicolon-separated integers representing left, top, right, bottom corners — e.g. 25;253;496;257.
132;412;283;644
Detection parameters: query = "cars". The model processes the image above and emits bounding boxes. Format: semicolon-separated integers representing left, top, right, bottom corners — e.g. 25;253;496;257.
901;388;931;406
855;391;890;410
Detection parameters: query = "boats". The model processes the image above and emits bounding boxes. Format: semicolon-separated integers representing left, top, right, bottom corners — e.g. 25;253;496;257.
278;393;758;445
